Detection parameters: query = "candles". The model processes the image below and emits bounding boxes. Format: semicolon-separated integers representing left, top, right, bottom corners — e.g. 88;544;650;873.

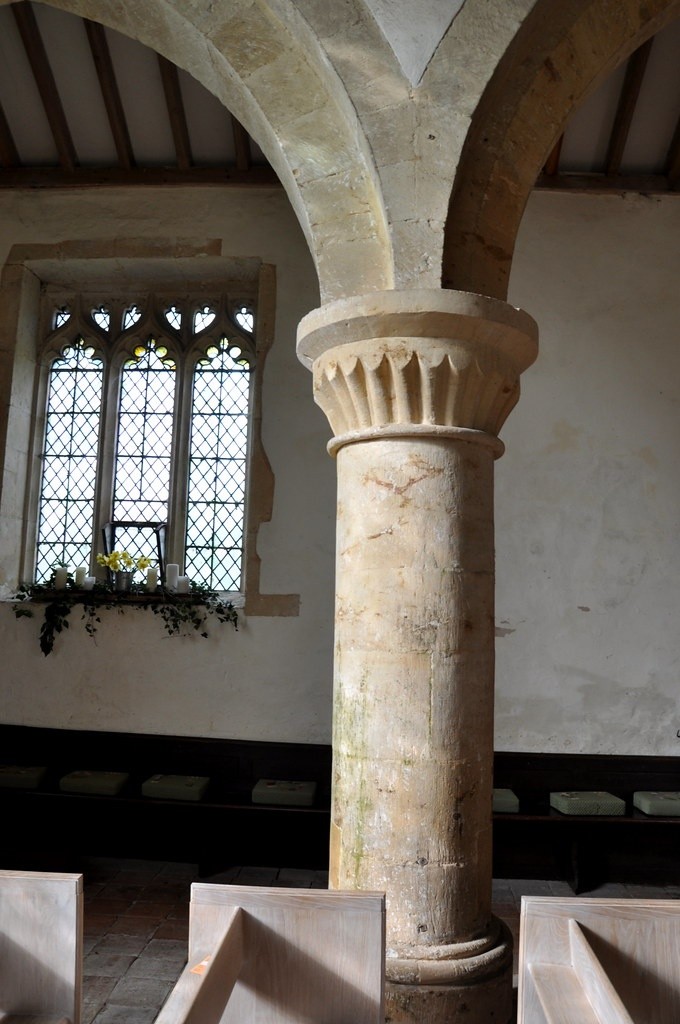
84;577;96;590
147;567;157;591
76;566;86;587
55;567;66;590
165;564;179;593
176;576;190;593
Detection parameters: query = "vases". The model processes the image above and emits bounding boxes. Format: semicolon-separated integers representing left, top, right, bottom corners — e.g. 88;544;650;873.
115;571;133;593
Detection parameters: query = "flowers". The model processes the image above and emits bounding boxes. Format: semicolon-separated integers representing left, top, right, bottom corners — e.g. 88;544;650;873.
96;550;152;572
